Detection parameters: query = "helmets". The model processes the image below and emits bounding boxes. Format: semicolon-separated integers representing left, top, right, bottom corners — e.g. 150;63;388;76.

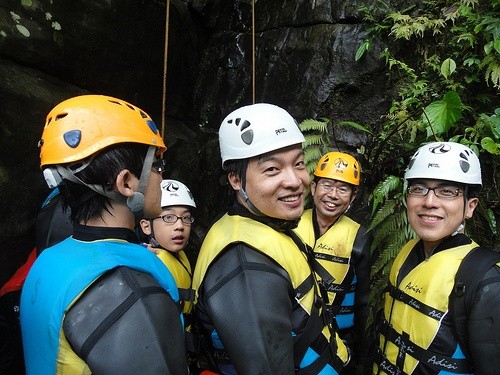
219;103;306;170
314;152;360;186
38;95;167;170
404;142;482;195
159;180;197;208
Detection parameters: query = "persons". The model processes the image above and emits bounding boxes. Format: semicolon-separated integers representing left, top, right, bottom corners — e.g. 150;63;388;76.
373;141;500;375
293;152;372;349
141;179;196;334
20;95;187;375
192;102;351;375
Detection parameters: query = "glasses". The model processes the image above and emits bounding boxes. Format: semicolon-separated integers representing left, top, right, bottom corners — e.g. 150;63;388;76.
154;214;195;224
315;182;353;195
408;183;465;198
107;159;165;187
